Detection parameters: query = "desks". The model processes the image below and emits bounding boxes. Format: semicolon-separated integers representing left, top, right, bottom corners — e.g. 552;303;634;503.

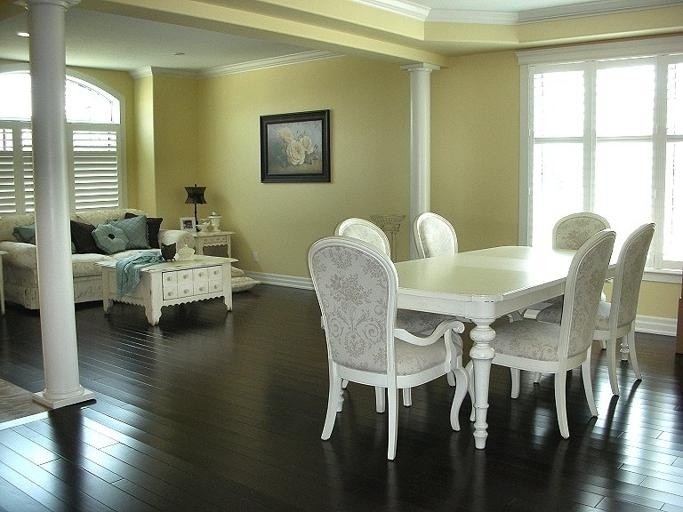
186;229;234;263
0;249;14;315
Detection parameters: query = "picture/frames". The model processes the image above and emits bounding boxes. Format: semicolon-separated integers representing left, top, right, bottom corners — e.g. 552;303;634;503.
179;216;198;231
257;107;331;186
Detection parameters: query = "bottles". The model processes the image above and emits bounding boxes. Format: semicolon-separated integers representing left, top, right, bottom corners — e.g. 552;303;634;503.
177;244;195;260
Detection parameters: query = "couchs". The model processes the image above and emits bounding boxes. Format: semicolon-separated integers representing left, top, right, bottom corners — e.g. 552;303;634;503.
95;255;238;326
0;206;197;312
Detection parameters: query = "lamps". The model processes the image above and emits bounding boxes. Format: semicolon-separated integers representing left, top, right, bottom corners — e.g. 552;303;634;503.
181;182;209;229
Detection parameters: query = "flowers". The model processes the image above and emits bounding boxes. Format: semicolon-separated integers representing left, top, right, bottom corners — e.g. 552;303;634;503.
270;125;320;171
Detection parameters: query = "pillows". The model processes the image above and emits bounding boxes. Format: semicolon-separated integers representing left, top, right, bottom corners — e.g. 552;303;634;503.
68;239;77;254
106;214;152;252
69;217;103;255
124;210;164;250
230;266;246;279
89;221;129;252
9;222;36;245
226;276;259;295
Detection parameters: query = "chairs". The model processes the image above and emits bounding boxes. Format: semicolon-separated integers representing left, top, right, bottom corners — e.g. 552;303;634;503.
412;209;522;402
329;218;458;408
487;228;619;439
304;234;462;461
531;219;656;400
541;213;616;353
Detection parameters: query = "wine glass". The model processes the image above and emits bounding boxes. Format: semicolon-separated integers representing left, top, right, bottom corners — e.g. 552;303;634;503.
207;215;222;232
